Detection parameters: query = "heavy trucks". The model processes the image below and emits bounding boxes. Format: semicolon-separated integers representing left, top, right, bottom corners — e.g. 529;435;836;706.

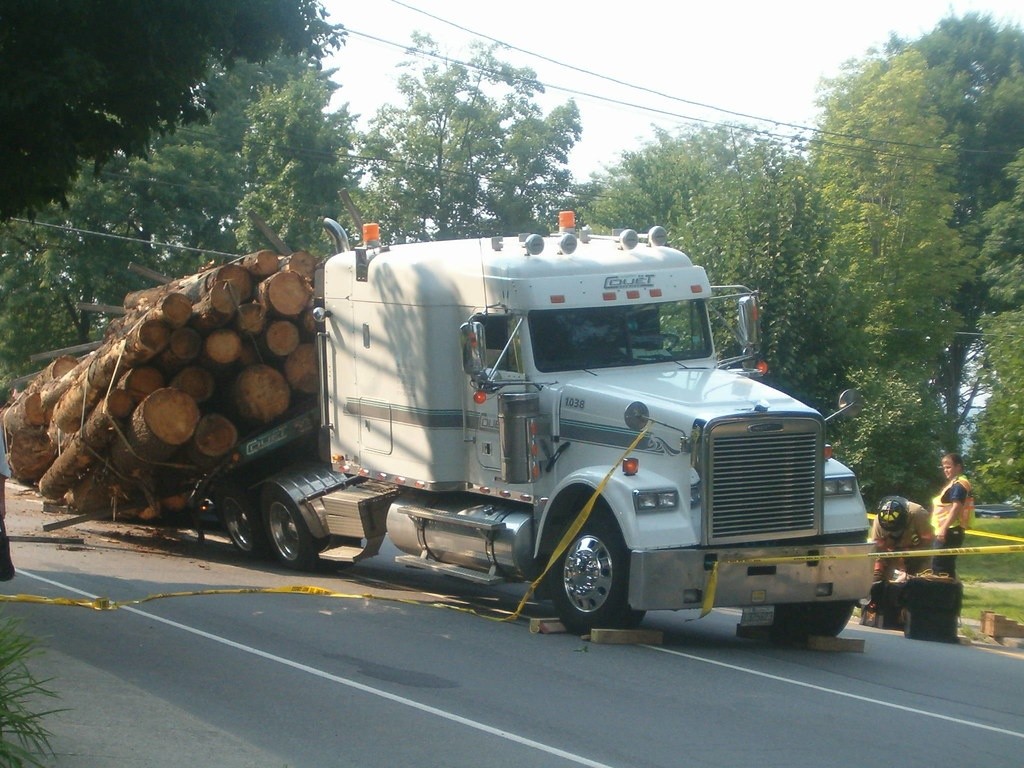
94;211;878;650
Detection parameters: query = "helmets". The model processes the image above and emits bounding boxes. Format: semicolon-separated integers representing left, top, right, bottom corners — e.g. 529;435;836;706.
878;496;910;531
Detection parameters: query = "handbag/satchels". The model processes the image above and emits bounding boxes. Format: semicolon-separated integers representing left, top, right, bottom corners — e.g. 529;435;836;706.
0;515;15;581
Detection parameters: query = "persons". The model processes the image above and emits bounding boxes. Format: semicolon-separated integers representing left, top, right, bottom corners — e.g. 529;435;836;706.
871;496;935;582
930;453;976;580
1;428;12;524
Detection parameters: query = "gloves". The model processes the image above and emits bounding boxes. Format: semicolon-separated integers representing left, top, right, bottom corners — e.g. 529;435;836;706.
904;556;924;574
